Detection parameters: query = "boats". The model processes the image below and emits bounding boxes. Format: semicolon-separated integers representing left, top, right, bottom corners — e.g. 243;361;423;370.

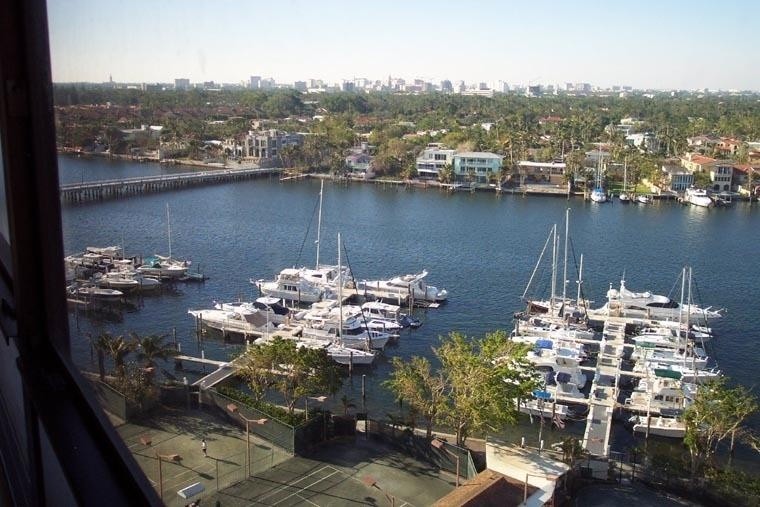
637;195;649;204
188;178;447;364
65;200;205;312
685;184;713;207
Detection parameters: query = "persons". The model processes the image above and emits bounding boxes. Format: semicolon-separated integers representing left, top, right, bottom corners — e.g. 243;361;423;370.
201;438;208;457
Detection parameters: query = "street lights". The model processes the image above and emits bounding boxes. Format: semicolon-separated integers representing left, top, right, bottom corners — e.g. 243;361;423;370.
138;366;155;417
363;475;395;506
139;434;183;501
523;472;560;506
430;438;459;489
227;403;268;479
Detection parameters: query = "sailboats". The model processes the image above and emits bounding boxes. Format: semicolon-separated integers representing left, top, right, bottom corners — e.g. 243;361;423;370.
620;156;630;202
591;141;608;203
490;206;724;438
